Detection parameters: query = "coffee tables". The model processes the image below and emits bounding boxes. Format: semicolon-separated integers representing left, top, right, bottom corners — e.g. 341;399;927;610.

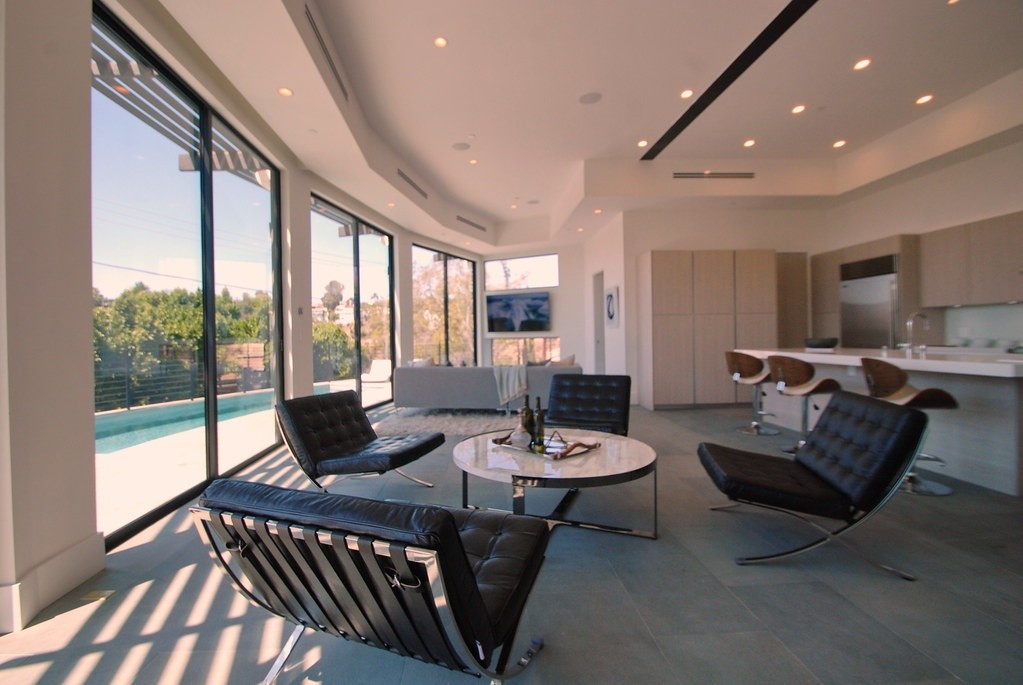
452;426;659;555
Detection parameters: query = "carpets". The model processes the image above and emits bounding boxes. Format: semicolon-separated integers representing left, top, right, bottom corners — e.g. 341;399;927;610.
371;405;521;438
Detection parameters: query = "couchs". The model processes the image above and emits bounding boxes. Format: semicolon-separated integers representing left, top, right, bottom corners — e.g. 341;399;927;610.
392;362;584;413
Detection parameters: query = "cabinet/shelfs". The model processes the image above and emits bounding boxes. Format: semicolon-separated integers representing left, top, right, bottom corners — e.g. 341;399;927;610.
918;210;1023;308
636;246;806;412
811;232;945;348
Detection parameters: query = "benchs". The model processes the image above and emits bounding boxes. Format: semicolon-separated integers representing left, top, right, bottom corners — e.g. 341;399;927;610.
189;476;553;685
273;390;445;493
698;390;929;583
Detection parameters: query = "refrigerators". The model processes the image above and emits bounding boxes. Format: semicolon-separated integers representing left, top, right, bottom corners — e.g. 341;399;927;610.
839;254;899;349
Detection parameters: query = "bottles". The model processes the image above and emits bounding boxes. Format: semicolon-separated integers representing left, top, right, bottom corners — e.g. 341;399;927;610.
522;394;534;449
530;396;545;454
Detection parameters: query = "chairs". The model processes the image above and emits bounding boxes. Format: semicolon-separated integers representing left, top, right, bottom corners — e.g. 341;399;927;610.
545;374;632;436
361;359;392;382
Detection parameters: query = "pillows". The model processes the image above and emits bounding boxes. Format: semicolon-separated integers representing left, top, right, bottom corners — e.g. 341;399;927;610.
416;357;438;368
522;354;577;367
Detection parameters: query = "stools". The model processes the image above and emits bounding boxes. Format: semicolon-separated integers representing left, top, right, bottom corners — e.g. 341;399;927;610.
860;357;959;497
724;351;782;435
768;356;841;453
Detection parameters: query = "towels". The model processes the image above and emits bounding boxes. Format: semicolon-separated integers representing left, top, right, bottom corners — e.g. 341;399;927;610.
493;364;528;407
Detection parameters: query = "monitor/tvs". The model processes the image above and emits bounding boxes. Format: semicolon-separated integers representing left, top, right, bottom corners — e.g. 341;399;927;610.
484;286;559;338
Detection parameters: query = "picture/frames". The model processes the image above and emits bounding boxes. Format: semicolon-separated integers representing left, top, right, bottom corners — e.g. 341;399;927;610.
605;286;619;327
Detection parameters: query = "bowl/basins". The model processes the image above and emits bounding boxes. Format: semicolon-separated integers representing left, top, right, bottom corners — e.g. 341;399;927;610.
804;337;838;348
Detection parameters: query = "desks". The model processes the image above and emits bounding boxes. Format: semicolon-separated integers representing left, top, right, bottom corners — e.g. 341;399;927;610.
734;348;1023;496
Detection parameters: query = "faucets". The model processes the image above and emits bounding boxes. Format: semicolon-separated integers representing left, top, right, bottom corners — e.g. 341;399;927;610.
906;311;931;350
